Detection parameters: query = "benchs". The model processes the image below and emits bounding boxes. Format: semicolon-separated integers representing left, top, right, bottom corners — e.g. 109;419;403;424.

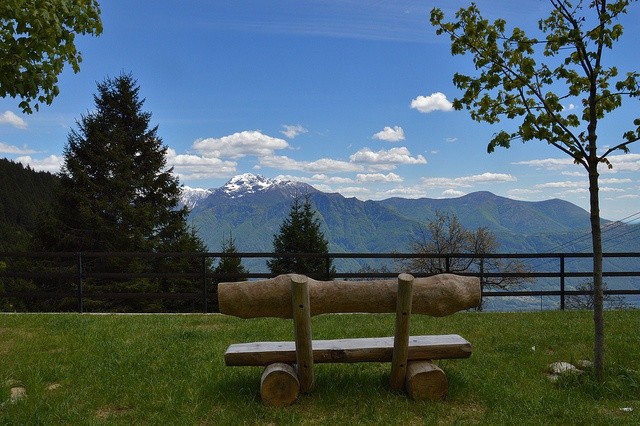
216;274;482;409
